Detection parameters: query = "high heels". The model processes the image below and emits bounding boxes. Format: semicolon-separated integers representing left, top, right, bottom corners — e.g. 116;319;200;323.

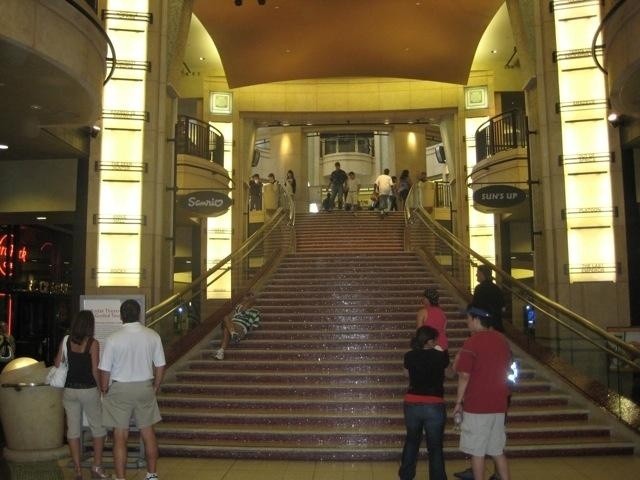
91;465;111;479
73;471;82;480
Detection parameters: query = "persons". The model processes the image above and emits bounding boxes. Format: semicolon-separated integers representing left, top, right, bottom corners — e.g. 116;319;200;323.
399;326;450;480
96;300;167;480
268;173;280;184
417;288;453;369
345;172;363;211
325;162;349;211
373;169;394;218
473;265;514;334
445;301;513;480
389;177;399;212
320;193;339;213
396;170;413;211
248;174;261;210
54;310;112;480
284;169;297;195
210;292;273;364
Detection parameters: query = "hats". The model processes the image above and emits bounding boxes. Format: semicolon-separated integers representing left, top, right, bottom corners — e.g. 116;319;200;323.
458;305;493;319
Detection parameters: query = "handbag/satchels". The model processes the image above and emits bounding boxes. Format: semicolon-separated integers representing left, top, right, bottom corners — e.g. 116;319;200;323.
46;334;69;387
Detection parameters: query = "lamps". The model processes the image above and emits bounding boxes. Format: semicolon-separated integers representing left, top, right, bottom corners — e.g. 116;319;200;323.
96;0;151;288
552;0;617;285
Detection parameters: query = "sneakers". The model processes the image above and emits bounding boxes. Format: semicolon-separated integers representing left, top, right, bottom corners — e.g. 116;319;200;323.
328;208;332;212
144;472;160;480
382;214;385;218
454;469;474;480
231;332;240;344
215;349;224;360
